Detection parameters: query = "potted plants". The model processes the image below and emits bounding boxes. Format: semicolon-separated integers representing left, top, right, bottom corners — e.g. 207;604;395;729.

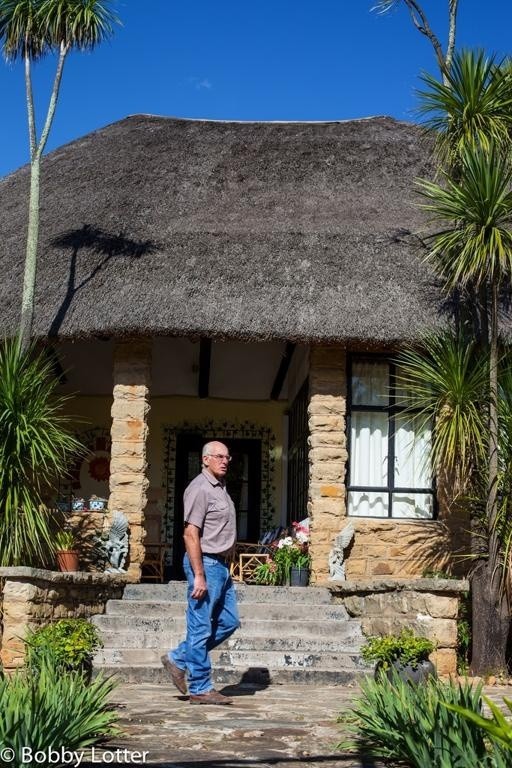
275;546;312;586
51;529;81;573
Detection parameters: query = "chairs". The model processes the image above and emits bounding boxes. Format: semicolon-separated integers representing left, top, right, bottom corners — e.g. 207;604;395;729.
224;526;289;584
138;513;169;583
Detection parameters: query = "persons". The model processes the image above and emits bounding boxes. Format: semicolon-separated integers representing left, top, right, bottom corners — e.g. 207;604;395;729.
160;440;250;706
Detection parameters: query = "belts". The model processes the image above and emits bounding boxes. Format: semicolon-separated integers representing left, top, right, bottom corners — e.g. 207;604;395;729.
202;553;226;563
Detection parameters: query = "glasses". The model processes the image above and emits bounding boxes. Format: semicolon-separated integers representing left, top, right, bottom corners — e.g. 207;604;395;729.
208;453;233;461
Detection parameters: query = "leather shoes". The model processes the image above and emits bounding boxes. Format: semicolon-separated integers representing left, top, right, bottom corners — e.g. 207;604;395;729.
189;688;234;705
161;654;188;695
